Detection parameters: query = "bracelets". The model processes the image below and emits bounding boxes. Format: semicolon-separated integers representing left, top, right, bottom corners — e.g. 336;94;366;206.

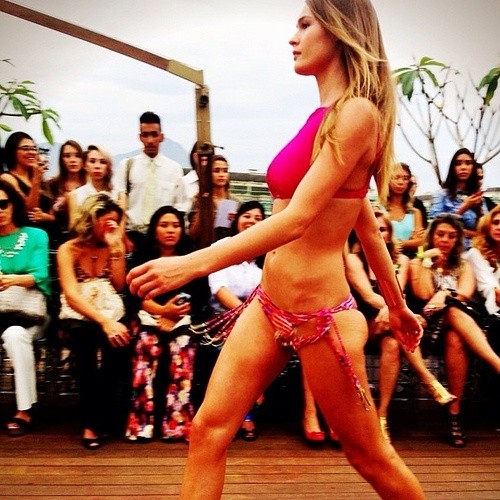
451;291;457;297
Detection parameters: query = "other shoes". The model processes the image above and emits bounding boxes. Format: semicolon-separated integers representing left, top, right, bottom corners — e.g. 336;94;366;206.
303;421;324;441
446;413;464;445
330;429;338;441
240;416;255;439
83;434;100;448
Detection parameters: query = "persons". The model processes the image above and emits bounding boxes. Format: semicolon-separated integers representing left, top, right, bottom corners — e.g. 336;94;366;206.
0;111;269;449
303;148;500;448
124;0;429;500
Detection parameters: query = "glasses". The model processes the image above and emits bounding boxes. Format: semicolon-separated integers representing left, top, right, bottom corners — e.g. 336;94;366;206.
0;198;11;210
17;146;37;151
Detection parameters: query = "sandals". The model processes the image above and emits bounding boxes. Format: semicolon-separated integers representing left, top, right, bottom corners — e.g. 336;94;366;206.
7;410;37;435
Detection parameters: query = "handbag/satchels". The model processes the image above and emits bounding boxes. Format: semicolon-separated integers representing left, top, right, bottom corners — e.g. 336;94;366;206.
59;277;125;322
0;284;47;317
